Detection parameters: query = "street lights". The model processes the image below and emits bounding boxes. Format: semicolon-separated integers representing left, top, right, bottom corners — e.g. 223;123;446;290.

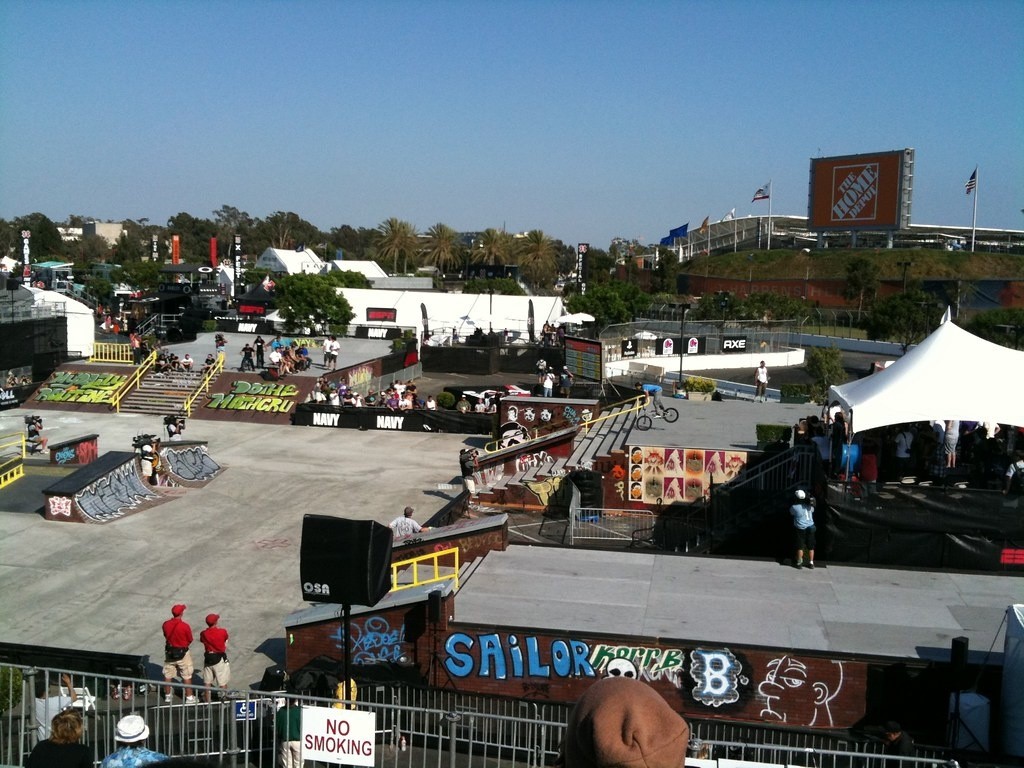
895;260;914;295
5;278;20;323
297;513;394;709
677;302;690;382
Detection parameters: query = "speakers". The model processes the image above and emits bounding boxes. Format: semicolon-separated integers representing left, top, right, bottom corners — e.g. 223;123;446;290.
427;591;442;622
300;514;394;606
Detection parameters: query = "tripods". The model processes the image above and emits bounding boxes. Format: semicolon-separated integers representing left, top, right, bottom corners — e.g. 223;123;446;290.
426;622;457;691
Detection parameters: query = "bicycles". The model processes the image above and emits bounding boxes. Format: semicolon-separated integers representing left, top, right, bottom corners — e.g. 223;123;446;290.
637;397;679;431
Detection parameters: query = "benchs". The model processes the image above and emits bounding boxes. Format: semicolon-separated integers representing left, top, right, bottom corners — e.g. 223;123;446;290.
264;361;279;381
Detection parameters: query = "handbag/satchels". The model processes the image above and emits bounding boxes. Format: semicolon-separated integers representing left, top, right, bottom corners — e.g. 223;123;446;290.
165;645;189;660
204;651;228;666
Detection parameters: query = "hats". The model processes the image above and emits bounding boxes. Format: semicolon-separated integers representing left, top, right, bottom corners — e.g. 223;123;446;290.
206;614;220;625
564;676;689;768
115;714;150;743
172;604;186;615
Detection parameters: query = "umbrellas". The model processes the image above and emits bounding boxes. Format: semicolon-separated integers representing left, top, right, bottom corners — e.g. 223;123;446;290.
635;330;657;340
557;311;595;329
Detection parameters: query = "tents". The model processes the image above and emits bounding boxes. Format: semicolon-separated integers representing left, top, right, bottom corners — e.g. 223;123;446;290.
827;305;1024;518
158;262;210;294
234;275;277;316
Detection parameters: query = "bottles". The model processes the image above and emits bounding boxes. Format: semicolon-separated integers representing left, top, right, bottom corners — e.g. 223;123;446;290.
401;736;407;752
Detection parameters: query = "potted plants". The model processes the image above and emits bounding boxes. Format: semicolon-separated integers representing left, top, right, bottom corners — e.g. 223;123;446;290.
780;383;806;404
681;374;717;401
755;424;792;451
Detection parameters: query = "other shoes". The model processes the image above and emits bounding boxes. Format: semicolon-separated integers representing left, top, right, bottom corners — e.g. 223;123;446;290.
186;696;200;704
165;694;173;702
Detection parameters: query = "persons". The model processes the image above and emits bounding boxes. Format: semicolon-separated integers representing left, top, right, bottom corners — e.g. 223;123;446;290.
789;412;1024;569
759;340;767;352
635;382;665;418
97;304;228;377
536;357;574;398
23;601;357;768
754;360;768;403
559;676;689;768
239;326;509;414
460;449;479;507
28;419;50;454
388;506;432;537
6;369;31;387
32;270;68;289
141;415;185;482
541;321;567;346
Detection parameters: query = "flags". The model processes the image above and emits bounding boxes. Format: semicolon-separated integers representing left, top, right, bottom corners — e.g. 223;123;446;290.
700;216;709;233
659;223;688;247
752;182;770;202
965;169;976;195
